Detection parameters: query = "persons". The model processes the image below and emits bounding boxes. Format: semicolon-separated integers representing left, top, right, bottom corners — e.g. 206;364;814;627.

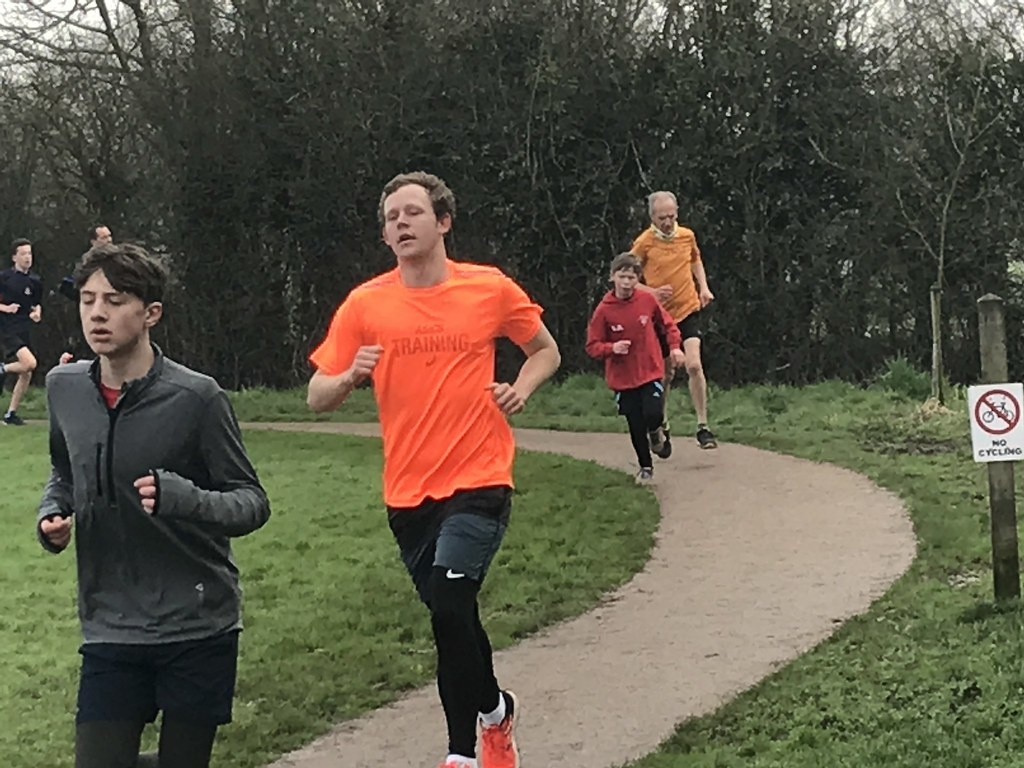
585;253;685;479
627;190;718;450
306;171;561;768
0;221;113;429
37;243;272;768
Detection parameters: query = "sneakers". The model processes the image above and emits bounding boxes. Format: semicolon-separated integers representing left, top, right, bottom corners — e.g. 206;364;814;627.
438;760;471;768
2;411;26;427
476;690;519;768
650;427;664;452
658;420;671;458
0;362;7;395
634;468;652;486
696;428;717;449
